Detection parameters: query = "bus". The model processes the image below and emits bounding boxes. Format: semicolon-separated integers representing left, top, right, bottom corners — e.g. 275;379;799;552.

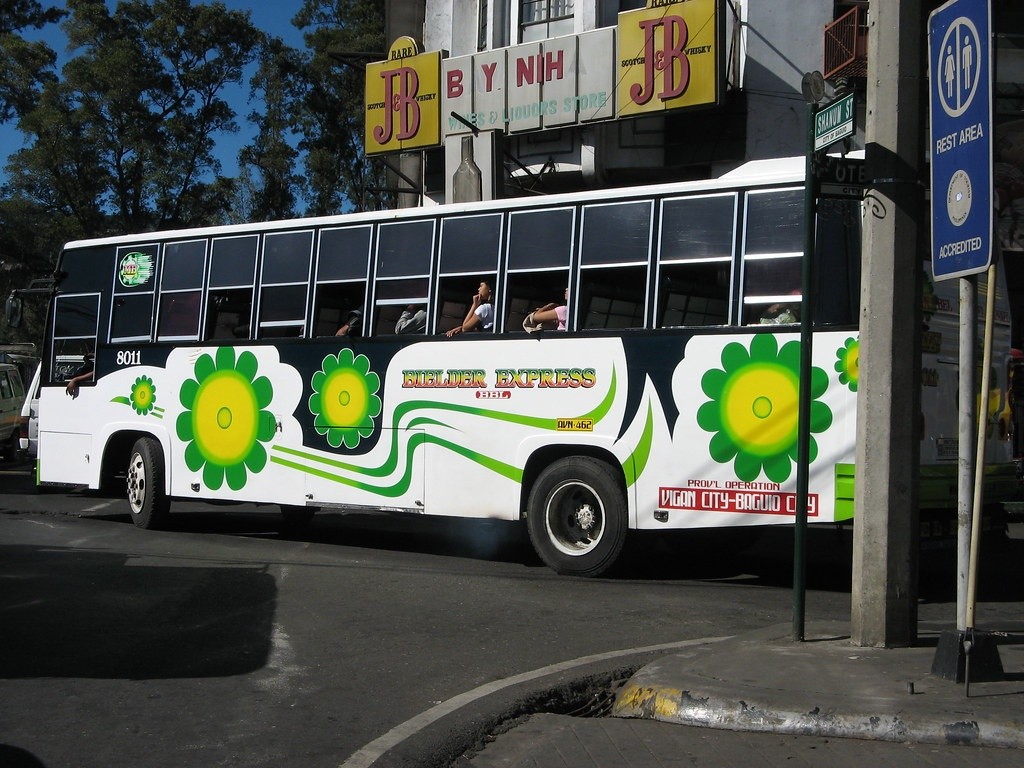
18;150;1023;580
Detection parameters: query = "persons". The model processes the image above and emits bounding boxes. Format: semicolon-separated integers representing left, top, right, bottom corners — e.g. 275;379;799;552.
334;304;365;337
394;289;426;335
64;353;95;396
757;288;803;324
534;284;569;331
445;277;494;337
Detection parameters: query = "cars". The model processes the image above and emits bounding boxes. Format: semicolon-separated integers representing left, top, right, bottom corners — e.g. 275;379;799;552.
0;343;94;465
